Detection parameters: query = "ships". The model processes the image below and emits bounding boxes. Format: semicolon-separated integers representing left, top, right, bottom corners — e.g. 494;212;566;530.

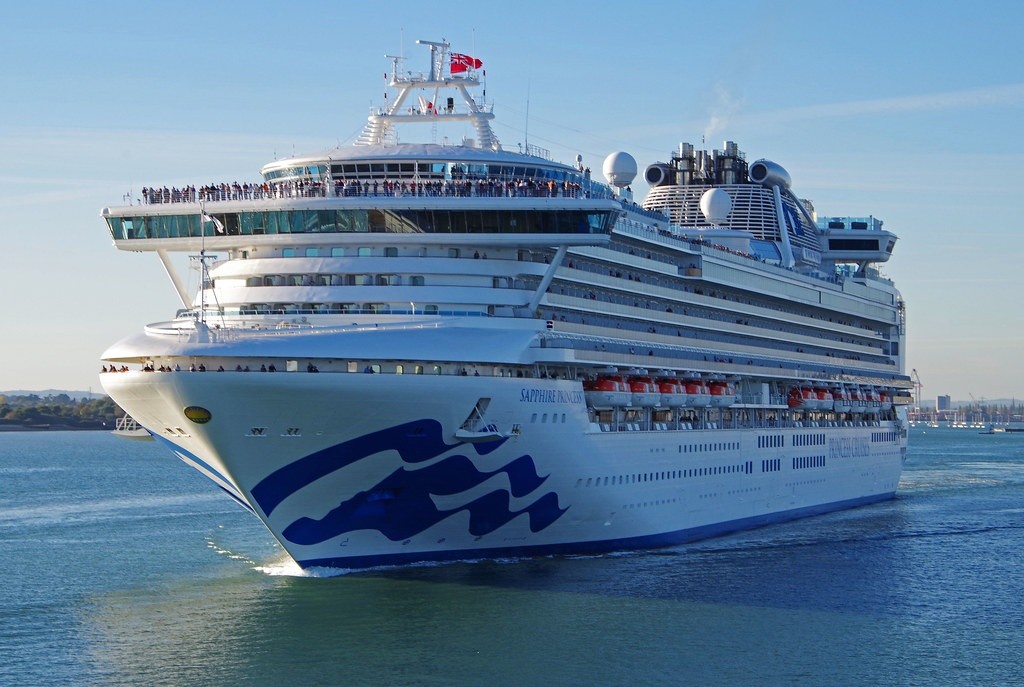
99;25;920;578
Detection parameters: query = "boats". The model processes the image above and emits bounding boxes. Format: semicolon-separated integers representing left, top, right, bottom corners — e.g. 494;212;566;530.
631;382;660;410
583;379;632;409
816;389;834;413
830;392;851;415
882;394;892;412
661;380;686;408
789;387;819;411
863;389;882;413
688;380;713;409
847;389;866;414
710;383;735;407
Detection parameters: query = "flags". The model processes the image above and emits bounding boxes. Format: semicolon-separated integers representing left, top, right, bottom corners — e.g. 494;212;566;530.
419;96;438;116
450;52;483;73
200;210;224;233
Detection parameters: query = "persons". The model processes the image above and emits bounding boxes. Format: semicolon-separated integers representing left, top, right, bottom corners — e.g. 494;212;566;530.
141;178;581;204
101;362;480;376
473;193;895;381
